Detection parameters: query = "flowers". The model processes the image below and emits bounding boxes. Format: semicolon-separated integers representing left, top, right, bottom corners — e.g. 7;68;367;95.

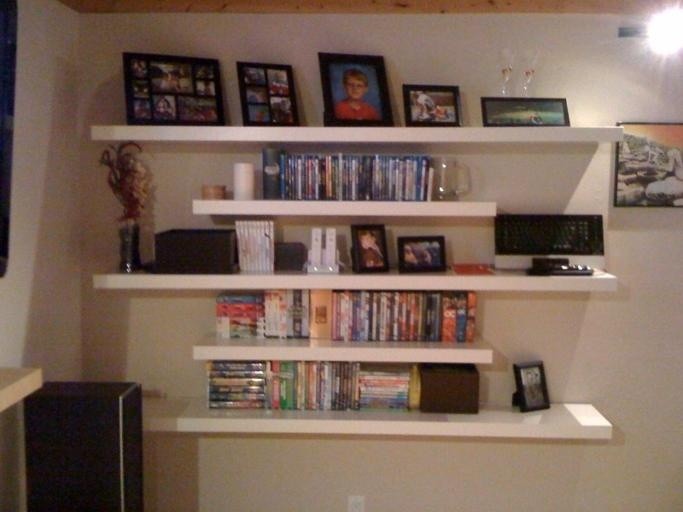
96;139;153;224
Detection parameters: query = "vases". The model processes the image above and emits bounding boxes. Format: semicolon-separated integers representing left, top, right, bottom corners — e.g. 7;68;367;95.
117;219;142;273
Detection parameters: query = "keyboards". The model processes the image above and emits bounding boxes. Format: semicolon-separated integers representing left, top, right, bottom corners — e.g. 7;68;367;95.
496;215;603;255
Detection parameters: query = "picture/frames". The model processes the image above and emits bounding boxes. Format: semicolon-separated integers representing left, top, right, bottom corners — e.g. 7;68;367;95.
121;50;224;126
479;94;570;127
349;222;390;273
613;120;683;209
511;359;549;412
315;50;396;126
236;60;299;127
400;82;463;126
395;233;447;274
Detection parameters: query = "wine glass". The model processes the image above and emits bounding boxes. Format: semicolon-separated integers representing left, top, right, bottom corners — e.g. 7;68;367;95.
496;48;511;98
517;49;539;98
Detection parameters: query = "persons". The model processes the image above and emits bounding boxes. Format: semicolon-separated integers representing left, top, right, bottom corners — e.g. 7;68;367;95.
335;68;381;120
529;113;543;125
415;89;456;121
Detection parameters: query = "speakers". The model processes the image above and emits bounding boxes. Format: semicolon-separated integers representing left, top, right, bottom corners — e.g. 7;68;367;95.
25;378;144;512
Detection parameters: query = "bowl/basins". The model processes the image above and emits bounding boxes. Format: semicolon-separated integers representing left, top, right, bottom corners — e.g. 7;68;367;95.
200;184;226;200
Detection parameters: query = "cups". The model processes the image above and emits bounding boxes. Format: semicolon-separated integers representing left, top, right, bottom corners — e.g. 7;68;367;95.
233;163;253;201
431;158;471;199
261;149;281;199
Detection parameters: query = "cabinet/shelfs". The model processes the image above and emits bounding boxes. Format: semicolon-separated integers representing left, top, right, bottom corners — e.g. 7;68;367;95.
88;124;630;440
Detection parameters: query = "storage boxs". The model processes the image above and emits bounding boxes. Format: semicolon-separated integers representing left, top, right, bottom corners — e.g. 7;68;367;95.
152;227;239;274
418;363;479;415
